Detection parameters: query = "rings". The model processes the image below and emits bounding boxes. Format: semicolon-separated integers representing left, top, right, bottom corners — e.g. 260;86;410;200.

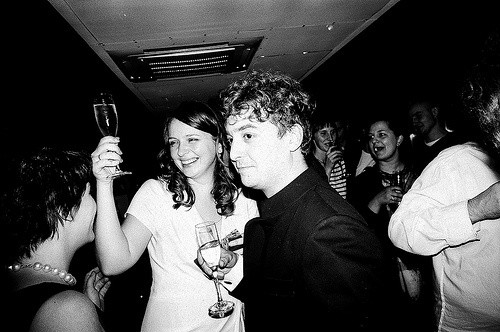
98;154;101;160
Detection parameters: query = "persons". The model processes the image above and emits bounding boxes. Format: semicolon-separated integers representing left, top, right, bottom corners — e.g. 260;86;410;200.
197;71;402;332
350;121;436;332
388;89;499;332
90;103;260;331
308;118;348;202
399;98;460;178
333;118;360;166
1;142;111;331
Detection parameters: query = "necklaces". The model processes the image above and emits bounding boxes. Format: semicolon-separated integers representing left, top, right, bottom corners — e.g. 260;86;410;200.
8;262;77;286
190;177;214;193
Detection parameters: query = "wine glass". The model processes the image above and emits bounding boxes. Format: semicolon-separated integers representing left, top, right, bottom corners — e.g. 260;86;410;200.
195;221;236;314
93;92;131;177
329;143;352;179
392;171;405;206
